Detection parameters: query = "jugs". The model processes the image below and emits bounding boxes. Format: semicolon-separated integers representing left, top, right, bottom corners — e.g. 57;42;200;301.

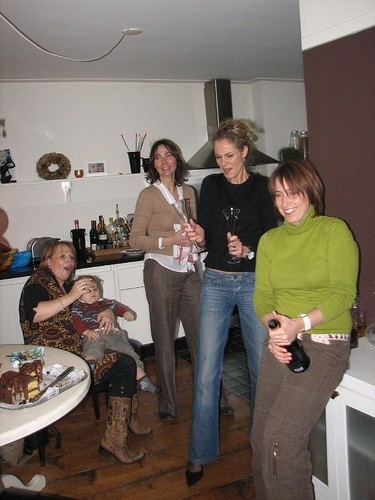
70;228;86;261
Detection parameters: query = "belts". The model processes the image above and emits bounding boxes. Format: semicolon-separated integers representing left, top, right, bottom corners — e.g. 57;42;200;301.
296;331;351;345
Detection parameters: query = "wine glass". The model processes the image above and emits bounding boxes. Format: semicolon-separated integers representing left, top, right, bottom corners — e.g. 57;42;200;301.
171;200;207;254
223;207;241;264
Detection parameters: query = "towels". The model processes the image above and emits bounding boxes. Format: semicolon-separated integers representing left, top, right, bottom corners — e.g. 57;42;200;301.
173;222;198;272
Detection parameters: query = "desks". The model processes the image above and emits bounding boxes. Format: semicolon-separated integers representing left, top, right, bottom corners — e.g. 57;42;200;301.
0;344;91;467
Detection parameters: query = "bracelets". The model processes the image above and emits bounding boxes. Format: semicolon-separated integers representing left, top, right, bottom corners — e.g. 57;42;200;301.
299;313;311;331
58;297;66;310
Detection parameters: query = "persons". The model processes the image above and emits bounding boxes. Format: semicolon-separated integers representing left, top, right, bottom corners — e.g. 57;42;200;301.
72;275;161;392
129;139;233;419
180;120;279;487
251;158;359;500
19;241;152;463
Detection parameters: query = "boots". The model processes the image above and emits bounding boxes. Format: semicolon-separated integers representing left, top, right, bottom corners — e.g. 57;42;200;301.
99;396;145;464
124;394;151;435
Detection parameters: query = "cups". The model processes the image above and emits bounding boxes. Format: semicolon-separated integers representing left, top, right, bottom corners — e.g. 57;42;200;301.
357;311;367;337
75;170;83;178
127;152;152;174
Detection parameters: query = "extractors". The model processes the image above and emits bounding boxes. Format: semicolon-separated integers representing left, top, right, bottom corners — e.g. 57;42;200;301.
182;77;280;168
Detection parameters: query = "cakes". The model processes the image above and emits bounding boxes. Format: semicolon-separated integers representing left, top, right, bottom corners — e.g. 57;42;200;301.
0;370;40;405
18;360;42;384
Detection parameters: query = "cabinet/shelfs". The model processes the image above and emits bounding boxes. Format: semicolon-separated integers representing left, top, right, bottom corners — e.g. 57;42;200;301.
0;260;187;359
309;386;375;500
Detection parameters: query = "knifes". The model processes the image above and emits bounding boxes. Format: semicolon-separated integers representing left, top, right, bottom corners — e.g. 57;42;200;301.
30;365;74;400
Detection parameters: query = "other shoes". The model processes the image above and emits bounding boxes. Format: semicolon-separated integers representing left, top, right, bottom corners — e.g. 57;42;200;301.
160;412;174;421
186;462;203;486
219;406;234;415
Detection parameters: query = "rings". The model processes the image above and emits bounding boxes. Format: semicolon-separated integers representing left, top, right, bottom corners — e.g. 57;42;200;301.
233;246;236;251
82;285;85;289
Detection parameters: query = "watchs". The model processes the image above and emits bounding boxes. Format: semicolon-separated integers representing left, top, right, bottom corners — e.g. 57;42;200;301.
243;244;255;260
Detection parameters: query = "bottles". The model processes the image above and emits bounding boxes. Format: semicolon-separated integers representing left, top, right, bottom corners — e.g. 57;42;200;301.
267;319;310;373
74;216;130;250
289;130;308;161
350;300;358;349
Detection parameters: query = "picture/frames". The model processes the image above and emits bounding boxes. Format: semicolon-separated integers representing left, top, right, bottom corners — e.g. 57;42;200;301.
85;161;108;177
